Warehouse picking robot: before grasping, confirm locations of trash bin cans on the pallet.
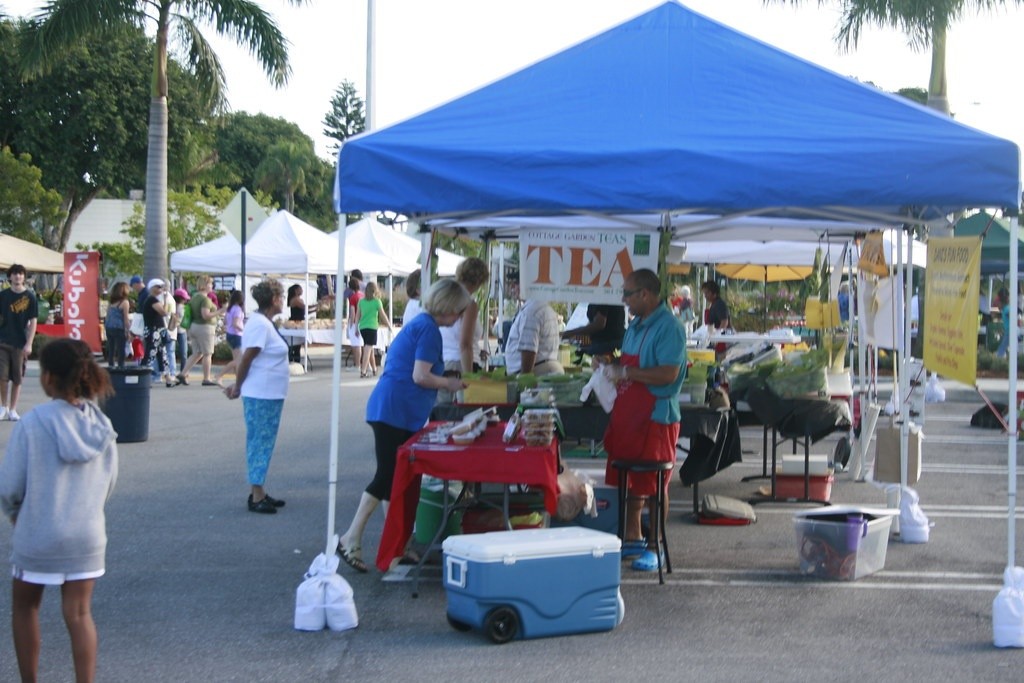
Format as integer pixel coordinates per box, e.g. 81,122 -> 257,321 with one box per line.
98,365 -> 155,443
415,472 -> 462,543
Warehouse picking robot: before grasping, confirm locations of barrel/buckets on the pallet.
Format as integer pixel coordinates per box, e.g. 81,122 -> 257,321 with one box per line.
415,479 -> 465,546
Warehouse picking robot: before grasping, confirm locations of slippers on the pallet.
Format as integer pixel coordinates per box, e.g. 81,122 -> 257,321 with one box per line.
337,540 -> 367,573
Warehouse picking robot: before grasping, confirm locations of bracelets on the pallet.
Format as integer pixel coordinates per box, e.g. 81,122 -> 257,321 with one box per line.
622,366 -> 627,379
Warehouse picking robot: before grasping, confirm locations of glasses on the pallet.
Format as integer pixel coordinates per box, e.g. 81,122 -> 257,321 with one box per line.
623,287 -> 643,297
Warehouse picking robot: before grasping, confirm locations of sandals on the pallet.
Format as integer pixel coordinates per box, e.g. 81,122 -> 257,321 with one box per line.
622,538 -> 647,559
632,548 -> 665,571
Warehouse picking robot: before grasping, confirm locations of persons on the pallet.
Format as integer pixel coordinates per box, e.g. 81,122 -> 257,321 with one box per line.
0,264 -> 39,421
0,338 -> 119,683
433,258 -> 488,407
504,272 -> 564,379
104,275 -> 306,387
223,279 -> 290,514
997,287 -> 1010,357
559,303 -> 626,364
702,281 -> 730,381
336,279 -> 471,572
403,269 -> 427,328
838,281 -> 849,322
591,269 -> 688,571
344,270 -> 366,298
355,282 -> 393,378
347,277 -> 365,371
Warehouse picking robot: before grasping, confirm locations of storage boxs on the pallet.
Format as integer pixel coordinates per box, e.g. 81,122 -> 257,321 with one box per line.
902,358 -> 925,413
873,424 -> 924,484
792,501 -> 902,581
442,525 -> 626,644
775,456 -> 835,503
553,458 -> 619,537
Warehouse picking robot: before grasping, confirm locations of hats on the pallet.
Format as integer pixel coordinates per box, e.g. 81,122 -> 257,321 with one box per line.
130,277 -> 141,286
148,279 -> 164,290
175,289 -> 191,300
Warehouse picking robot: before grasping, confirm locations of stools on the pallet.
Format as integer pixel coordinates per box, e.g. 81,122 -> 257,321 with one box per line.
610,457 -> 674,584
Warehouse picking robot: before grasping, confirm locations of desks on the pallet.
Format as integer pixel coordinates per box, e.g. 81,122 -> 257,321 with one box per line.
684,334 -> 801,343
379,423 -> 558,598
743,392 -> 851,506
279,326 -> 403,368
429,402 -> 742,514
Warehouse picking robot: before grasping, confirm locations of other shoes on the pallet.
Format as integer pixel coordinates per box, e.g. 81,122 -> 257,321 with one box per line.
0,407 -> 10,420
201,382 -> 215,385
248,495 -> 277,514
373,370 -> 378,375
176,374 -> 188,385
8,410 -> 20,420
166,380 -> 177,388
361,373 -> 369,378
261,494 -> 285,506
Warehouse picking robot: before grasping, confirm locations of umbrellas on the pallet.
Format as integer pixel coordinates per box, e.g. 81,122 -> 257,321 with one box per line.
713,263 -> 813,332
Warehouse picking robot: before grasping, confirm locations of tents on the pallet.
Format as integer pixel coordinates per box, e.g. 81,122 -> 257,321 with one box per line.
170,210 -> 468,373
915,212 -> 1024,349
326,0 -> 1020,567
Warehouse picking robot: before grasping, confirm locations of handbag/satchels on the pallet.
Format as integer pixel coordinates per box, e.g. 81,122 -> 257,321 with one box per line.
125,342 -> 134,358
131,336 -> 144,360
181,304 -> 191,330
